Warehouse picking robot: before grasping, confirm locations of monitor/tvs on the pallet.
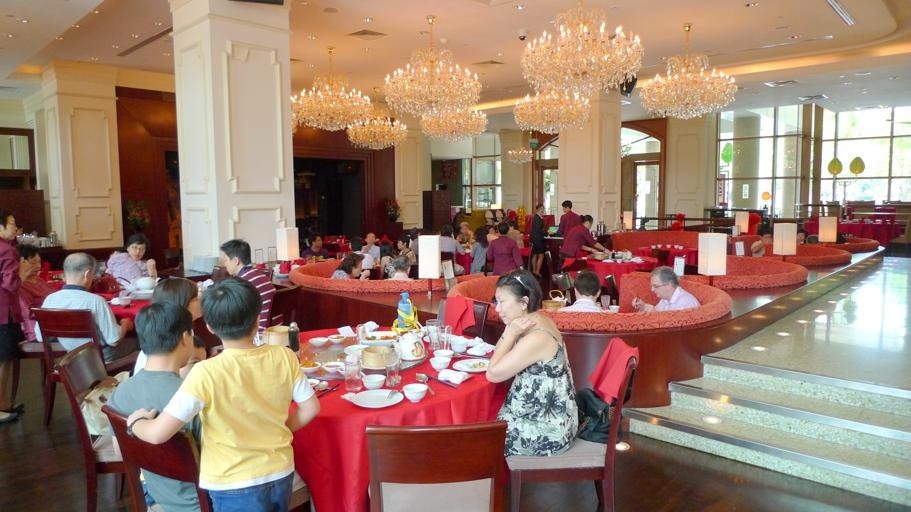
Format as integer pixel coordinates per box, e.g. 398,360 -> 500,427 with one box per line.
548,226 -> 559,235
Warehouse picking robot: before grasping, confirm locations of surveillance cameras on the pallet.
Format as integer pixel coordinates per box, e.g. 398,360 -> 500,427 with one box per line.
519,29 -> 528,40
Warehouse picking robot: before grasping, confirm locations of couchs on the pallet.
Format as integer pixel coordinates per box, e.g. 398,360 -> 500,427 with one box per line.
289,231 -> 880,334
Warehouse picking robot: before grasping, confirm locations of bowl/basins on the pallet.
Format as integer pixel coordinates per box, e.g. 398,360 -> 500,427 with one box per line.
329,334 -> 348,343
402,384 -> 429,403
309,338 -> 329,346
433,350 -> 456,361
430,357 -> 450,369
301,361 -> 345,376
362,374 -> 384,388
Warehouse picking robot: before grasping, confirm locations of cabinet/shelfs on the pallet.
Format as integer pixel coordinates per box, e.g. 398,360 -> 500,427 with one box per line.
423,191 -> 451,230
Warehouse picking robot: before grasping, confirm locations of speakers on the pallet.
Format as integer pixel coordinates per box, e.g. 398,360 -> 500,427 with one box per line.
620,76 -> 637,96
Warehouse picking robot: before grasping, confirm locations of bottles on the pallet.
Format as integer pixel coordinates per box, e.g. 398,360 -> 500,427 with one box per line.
18,231 -> 58,245
398,292 -> 416,328
288,322 -> 301,351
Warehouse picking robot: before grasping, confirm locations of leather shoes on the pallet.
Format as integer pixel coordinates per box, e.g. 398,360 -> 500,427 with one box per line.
0,401 -> 26,423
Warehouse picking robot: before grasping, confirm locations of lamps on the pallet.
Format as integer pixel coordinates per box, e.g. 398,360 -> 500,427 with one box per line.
828,157 -> 865,217
511,2 -> 645,135
638,23 -> 739,121
719,143 -> 733,169
382,15 -> 489,143
292,47 -> 406,150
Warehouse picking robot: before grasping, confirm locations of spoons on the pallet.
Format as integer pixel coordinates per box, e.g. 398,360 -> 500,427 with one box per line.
416,372 -> 437,396
314,381 -> 329,394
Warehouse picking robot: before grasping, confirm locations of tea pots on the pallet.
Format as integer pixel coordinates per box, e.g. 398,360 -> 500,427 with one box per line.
134,276 -> 161,289
550,289 -> 568,306
393,330 -> 427,360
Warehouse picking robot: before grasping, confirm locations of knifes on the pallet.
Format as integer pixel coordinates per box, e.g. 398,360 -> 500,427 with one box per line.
431,375 -> 458,388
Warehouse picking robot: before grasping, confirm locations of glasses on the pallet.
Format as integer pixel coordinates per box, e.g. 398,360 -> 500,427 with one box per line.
650,282 -> 669,289
498,271 -> 530,293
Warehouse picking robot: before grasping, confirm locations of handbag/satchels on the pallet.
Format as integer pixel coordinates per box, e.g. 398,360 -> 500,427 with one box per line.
576,387 -> 624,444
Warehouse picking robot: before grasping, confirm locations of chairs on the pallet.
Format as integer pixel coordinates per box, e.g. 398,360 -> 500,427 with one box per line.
504,337 -> 637,512
437,297 -> 490,339
365,420 -> 508,512
54,340 -> 126,512
27,306 -> 138,437
99,403 -> 312,512
259,267 -> 302,328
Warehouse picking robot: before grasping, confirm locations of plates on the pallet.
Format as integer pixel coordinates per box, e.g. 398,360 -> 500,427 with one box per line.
451,358 -> 491,374
308,378 -> 320,388
353,390 -> 403,409
345,331 -> 400,370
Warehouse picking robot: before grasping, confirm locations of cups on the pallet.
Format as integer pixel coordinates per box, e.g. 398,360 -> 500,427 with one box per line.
425,318 -> 494,355
255,332 -> 270,347
111,290 -> 154,306
596,249 -> 646,264
384,347 -> 403,387
597,295 -> 621,313
345,361 -> 363,390
